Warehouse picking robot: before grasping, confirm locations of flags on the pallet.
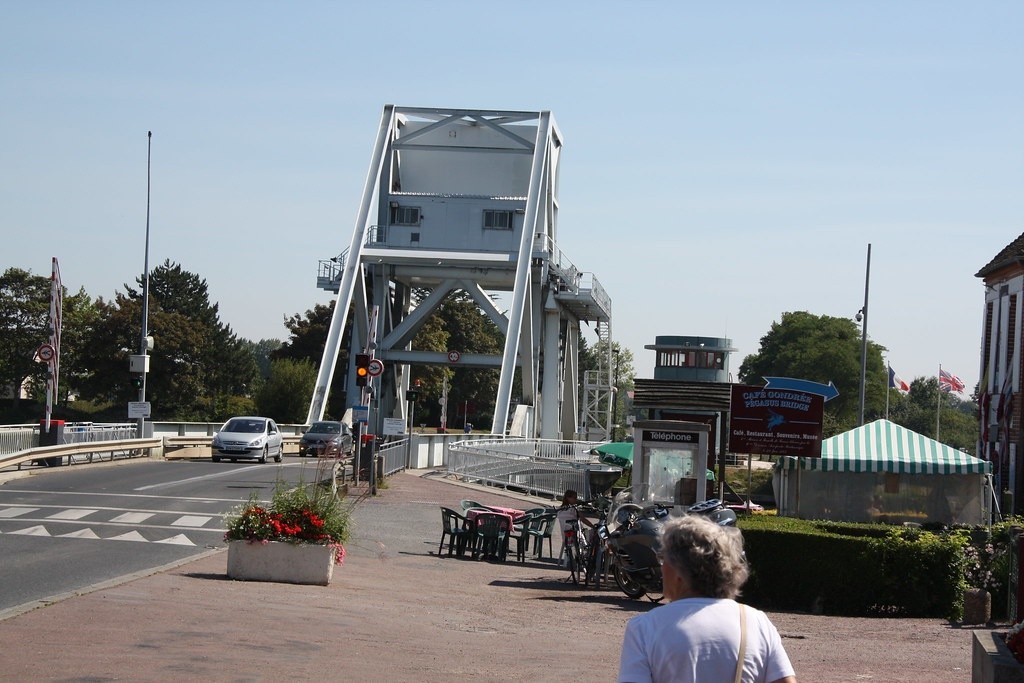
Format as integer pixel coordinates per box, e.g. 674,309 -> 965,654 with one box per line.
940,369 -> 966,394
888,365 -> 909,391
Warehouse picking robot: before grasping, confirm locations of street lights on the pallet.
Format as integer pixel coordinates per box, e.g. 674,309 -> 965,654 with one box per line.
855,244 -> 873,426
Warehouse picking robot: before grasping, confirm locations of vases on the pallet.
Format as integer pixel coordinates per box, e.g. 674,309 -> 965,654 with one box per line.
961,588 -> 991,623
228,539 -> 335,586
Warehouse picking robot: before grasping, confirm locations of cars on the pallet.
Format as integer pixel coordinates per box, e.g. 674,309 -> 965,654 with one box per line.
299,421 -> 353,457
211,416 -> 284,464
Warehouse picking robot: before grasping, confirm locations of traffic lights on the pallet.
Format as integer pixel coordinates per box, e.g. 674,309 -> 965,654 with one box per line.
355,354 -> 370,386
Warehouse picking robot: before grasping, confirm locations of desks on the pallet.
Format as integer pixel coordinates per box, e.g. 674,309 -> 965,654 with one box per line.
468,510 -> 514,557
485,505 -> 526,553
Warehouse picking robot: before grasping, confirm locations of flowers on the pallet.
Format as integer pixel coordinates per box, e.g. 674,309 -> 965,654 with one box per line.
959,543 -> 1009,593
218,447 -> 391,567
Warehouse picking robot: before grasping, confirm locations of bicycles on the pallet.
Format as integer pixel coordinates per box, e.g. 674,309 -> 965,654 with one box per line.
564,519 -> 589,586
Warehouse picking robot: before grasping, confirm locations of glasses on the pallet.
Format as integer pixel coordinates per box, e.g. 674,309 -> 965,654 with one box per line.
656,553 -> 665,565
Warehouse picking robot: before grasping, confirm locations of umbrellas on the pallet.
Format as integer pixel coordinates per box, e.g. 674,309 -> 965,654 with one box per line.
592,441 -> 716,482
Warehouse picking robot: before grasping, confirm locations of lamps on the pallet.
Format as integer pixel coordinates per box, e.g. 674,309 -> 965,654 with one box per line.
515,209 -> 524,214
390,201 -> 399,209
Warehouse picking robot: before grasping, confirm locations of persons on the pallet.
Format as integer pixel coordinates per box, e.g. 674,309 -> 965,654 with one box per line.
557,490 -> 597,585
855,493 -> 884,523
619,513 -> 798,683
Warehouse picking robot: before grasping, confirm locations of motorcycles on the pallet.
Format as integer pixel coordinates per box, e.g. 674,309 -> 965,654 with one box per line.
595,485 -> 738,599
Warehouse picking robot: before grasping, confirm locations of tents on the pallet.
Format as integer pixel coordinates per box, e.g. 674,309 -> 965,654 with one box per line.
771,418 -> 1005,539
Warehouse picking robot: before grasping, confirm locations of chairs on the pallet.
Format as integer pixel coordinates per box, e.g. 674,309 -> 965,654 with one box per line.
438,498 -> 561,564
